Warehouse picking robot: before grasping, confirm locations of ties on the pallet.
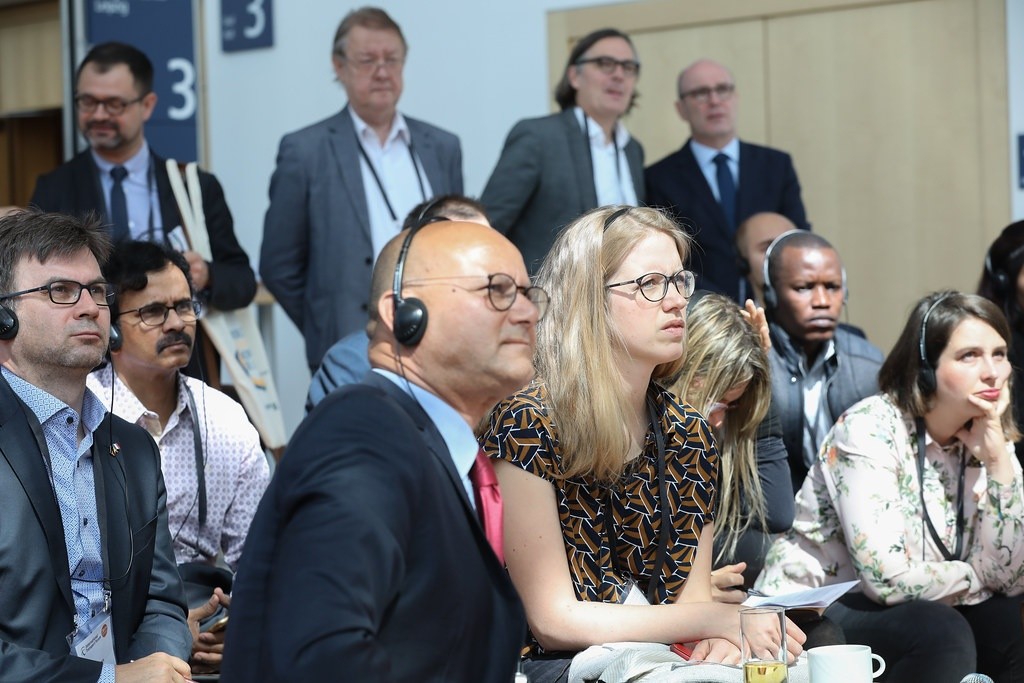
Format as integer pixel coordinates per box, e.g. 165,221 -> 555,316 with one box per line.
471,445 -> 505,570
110,167 -> 132,247
711,154 -> 736,243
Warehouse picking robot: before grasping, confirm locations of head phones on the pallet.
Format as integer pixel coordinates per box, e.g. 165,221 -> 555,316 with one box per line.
109,324 -> 123,351
763,229 -> 850,306
917,295 -> 954,395
392,215 -> 450,346
0,304 -> 19,340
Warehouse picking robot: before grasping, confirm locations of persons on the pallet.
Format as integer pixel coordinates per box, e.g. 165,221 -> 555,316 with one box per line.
86,240 -> 270,674
303,194 -> 489,420
643,60 -> 811,308
478,205 -> 807,683
259,7 -> 463,378
219,222 -> 551,683
478,29 -> 647,284
0,206 -> 203,683
650,210 -> 1024,682
32,41 -> 257,386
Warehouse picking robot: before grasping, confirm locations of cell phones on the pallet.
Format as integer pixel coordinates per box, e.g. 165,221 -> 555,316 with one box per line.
207,616 -> 229,633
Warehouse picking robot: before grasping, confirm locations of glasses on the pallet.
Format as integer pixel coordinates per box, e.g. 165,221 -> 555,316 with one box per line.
681,83 -> 735,101
605,270 -> 697,303
0,280 -> 117,306
405,273 -> 551,323
74,92 -> 144,116
343,55 -> 403,78
118,300 -> 205,326
577,56 -> 641,78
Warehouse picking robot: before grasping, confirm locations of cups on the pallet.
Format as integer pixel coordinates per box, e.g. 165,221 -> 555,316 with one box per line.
738,606 -> 789,682
807,644 -> 885,683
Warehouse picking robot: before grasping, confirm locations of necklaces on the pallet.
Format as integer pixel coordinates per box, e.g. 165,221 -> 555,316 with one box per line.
915,416 -> 973,562
